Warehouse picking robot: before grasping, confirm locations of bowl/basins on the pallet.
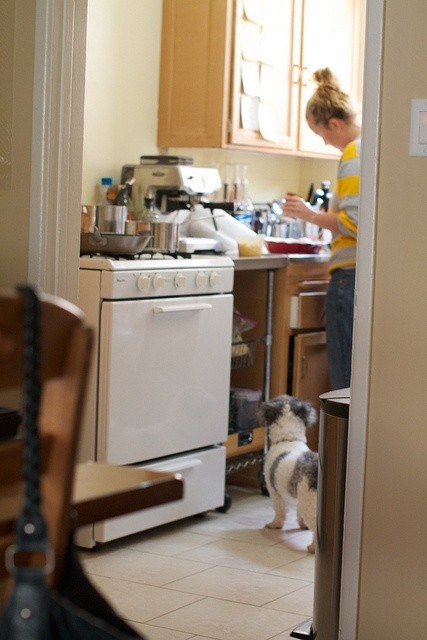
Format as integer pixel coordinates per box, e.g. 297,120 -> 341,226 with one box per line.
236,232 -> 264,257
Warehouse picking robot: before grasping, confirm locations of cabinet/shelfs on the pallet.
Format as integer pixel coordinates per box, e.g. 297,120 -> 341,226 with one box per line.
233,256 -> 335,487
157,1 -> 366,162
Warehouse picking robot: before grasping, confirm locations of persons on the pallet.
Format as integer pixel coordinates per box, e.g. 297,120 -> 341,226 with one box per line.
280,64 -> 362,390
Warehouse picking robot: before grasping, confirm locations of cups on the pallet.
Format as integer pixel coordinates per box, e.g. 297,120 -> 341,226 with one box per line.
98,205 -> 127,234
153,222 -> 179,252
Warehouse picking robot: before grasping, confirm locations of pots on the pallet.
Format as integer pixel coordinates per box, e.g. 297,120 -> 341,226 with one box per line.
81,227 -> 153,256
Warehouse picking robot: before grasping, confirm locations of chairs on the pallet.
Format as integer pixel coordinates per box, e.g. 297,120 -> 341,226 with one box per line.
2,285 -> 95,638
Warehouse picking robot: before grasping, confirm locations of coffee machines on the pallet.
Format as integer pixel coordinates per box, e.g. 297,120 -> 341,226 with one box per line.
121,155 -> 224,255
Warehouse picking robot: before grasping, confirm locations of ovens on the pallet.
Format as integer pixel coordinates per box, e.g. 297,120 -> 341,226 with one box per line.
77,292 -> 235,549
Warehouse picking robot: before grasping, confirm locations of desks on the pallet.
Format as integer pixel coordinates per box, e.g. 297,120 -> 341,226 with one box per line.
0,459 -> 182,640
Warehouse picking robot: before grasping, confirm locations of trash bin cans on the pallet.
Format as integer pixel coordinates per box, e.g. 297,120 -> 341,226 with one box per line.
307,388 -> 351,640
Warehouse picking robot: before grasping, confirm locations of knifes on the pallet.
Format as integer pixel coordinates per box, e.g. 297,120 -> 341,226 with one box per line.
305,178 -> 329,211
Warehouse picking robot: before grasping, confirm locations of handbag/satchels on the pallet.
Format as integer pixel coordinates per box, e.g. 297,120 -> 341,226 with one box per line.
0,540 -> 143,640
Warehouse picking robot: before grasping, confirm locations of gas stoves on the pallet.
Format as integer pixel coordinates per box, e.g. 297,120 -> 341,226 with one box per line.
78,250 -> 236,298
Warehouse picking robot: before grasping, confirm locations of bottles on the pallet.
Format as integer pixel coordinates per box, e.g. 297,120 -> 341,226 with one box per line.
232,164 -> 254,232
96,176 -> 117,205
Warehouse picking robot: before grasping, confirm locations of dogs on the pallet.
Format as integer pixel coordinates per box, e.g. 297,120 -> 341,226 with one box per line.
259,394 -> 321,555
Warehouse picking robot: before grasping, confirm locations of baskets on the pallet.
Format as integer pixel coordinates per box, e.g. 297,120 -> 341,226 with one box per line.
231,339 -> 255,369
